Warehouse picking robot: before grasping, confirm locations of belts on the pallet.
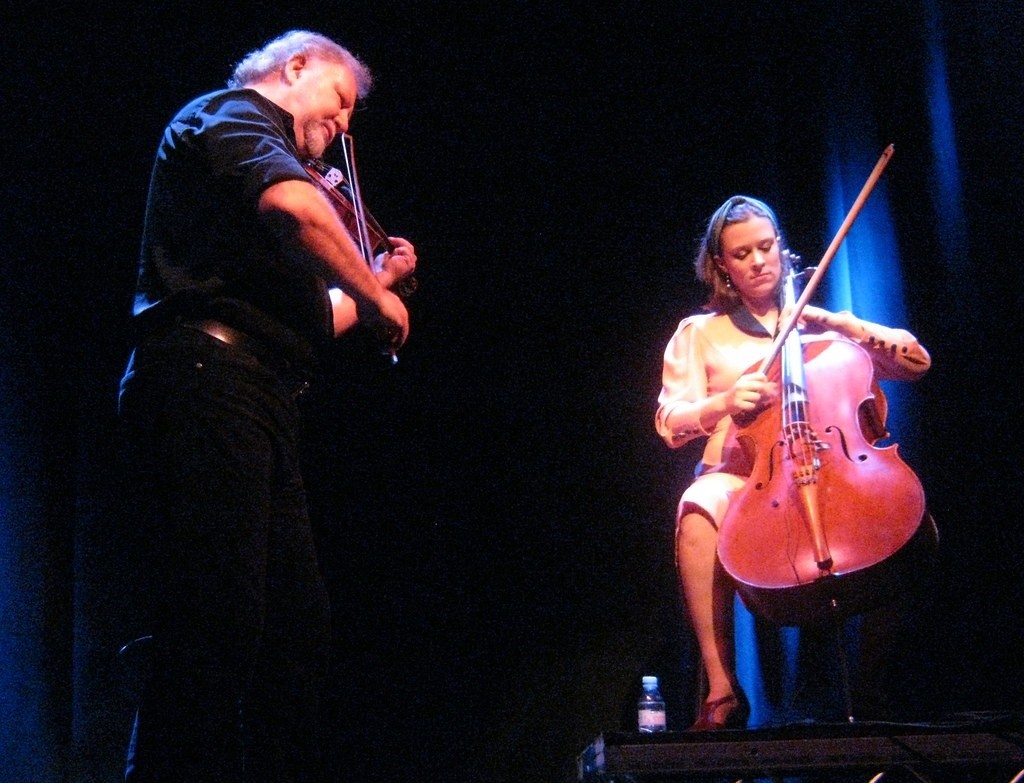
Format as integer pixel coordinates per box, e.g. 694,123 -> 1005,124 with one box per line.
186,319 -> 310,401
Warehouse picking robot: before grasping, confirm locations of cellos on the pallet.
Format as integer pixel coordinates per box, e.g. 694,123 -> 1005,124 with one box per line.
713,237 -> 939,721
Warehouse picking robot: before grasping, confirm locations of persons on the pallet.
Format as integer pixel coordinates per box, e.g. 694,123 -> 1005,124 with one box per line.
116,28 -> 417,783
655,195 -> 931,733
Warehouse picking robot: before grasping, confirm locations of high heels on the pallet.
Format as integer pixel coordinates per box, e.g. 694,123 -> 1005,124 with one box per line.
683,688 -> 751,732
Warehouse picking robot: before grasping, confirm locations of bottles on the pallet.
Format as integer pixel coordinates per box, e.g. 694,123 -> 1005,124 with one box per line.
637,676 -> 666,733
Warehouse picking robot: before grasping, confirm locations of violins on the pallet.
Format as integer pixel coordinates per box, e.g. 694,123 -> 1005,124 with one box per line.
306,162 -> 419,296
